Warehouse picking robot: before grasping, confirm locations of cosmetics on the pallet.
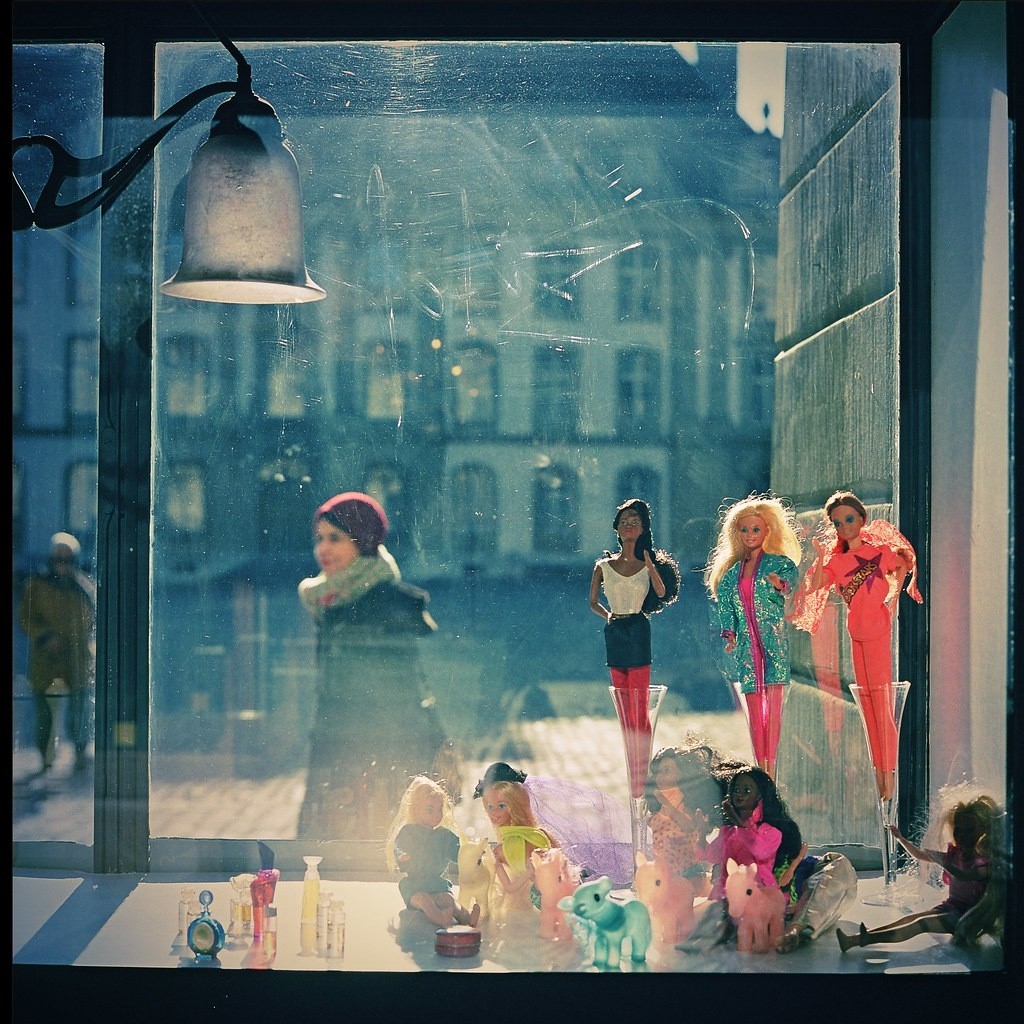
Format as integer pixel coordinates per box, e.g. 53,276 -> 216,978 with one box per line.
178,855 -> 346,959
435,925 -> 481,958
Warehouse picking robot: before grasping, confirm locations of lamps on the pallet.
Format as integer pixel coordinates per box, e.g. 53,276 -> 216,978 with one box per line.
1,1 -> 326,305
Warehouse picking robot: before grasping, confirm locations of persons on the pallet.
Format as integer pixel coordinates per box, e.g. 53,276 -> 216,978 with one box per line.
834,795 -> 1005,953
703,494 -> 802,782
786,492 -> 924,802
473,763 -> 561,910
297,492 -> 438,839
386,776 -> 480,928
587,498 -> 681,797
645,746 -> 857,954
21,532 -> 96,771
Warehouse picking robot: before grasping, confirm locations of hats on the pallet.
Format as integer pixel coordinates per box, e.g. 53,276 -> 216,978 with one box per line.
315,493 -> 388,556
